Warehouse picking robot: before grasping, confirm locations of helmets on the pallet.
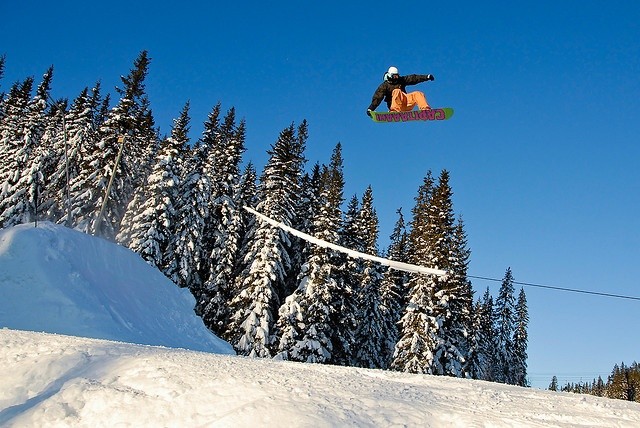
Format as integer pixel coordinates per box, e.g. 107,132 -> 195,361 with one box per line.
388,66 -> 398,75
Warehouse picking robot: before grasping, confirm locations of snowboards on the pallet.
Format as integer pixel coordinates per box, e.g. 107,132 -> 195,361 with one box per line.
366,108 -> 454,122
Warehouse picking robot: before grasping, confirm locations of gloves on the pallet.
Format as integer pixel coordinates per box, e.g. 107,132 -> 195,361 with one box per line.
428,74 -> 434,80
367,109 -> 372,116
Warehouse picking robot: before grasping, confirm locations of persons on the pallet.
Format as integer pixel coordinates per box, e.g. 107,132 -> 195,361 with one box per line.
366,65 -> 434,116
